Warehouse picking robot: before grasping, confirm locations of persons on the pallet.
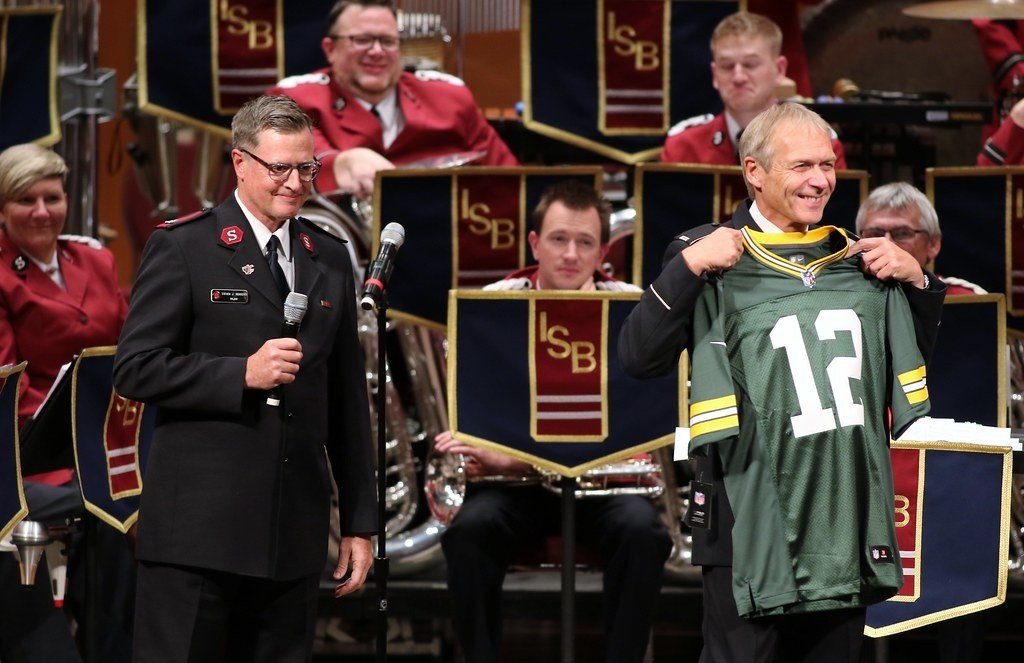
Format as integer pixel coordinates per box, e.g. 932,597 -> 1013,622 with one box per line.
971,18 -> 1024,569
434,187 -> 675,663
857,183 -> 987,295
0,143 -> 136,663
264,0 -> 520,526
620,102 -> 946,663
661,12 -> 848,170
112,95 -> 377,663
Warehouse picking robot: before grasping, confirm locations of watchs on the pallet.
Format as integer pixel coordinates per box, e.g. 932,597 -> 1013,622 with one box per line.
922,275 -> 930,290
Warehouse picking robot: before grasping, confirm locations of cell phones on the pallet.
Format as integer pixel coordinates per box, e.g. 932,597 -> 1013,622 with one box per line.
360,221 -> 405,310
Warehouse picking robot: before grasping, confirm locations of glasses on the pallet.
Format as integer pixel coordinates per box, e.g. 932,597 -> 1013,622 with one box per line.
859,226 -> 930,244
241,148 -> 322,182
329,34 -> 403,53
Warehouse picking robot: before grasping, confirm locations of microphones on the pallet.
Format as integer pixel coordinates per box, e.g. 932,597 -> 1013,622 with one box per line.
265,292 -> 308,406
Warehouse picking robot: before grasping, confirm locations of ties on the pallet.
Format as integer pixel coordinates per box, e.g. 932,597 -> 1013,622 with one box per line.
737,129 -> 744,142
266,235 -> 290,303
370,108 -> 379,120
